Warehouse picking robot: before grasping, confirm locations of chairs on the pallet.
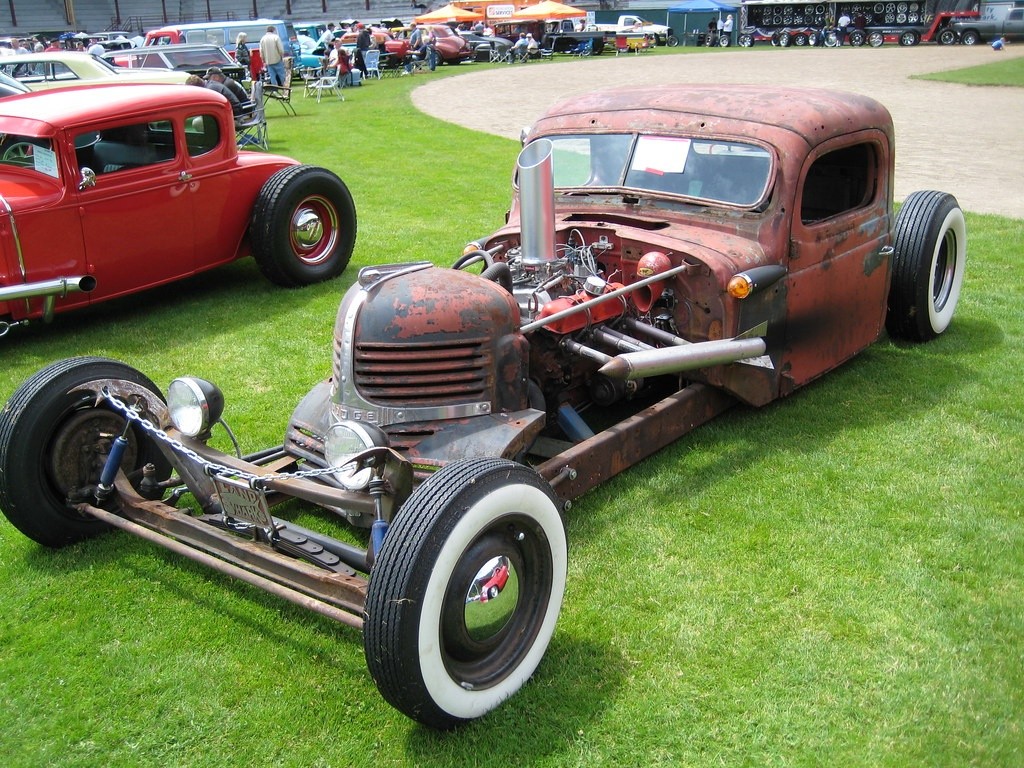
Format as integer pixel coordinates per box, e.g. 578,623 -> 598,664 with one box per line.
615,35 -> 632,54
300,43 -> 433,104
488,36 -> 556,64
263,57 -> 297,116
91,141 -> 156,173
570,39 -> 585,58
234,80 -> 269,152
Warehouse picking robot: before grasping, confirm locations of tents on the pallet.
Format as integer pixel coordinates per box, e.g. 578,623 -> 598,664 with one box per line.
60,31 -> 107,46
414,5 -> 483,27
512,1 -> 587,30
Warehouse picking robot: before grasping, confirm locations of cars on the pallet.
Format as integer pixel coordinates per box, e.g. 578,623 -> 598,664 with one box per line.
0,79 -> 967,727
0,82 -> 357,329
0,16 -> 514,98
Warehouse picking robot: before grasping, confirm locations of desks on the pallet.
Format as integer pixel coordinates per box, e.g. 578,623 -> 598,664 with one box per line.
406,50 -> 422,71
379,51 -> 397,77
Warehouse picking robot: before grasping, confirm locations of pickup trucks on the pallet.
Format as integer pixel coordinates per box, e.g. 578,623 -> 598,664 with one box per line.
953,7 -> 1024,45
545,19 -> 617,55
587,15 -> 671,44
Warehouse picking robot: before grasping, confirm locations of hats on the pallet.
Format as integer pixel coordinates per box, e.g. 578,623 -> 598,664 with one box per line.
50,38 -> 58,43
202,66 -> 222,78
328,23 -> 336,27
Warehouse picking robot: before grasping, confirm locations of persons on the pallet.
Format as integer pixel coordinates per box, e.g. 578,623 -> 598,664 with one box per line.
0,38 -> 104,76
401,22 -> 427,75
642,33 -> 660,46
992,37 -> 1005,50
185,67 -> 252,120
508,33 -> 538,64
575,20 -> 585,32
235,26 -> 286,94
458,20 -> 493,38
819,12 -> 866,46
708,15 -> 734,47
514,22 -> 540,36
427,27 -> 436,71
312,23 -> 377,88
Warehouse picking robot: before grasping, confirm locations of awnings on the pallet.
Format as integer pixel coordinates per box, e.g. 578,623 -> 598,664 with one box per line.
666,0 -> 738,48
